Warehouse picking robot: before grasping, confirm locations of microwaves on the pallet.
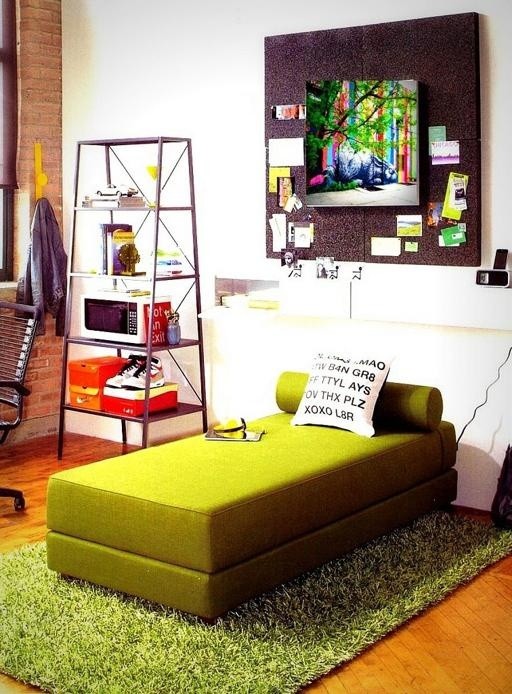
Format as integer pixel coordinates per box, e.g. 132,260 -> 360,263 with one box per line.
78,291 -> 170,345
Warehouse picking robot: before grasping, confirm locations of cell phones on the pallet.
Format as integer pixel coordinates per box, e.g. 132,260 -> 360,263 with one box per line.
493,249 -> 508,269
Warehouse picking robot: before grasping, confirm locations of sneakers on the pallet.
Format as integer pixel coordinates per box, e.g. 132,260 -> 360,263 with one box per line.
122,356 -> 167,390
105,355 -> 148,388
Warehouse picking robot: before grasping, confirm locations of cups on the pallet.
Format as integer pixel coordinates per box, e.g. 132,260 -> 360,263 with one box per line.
167,324 -> 180,344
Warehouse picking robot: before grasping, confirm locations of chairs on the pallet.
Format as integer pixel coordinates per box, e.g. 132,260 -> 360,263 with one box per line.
0,300 -> 40,516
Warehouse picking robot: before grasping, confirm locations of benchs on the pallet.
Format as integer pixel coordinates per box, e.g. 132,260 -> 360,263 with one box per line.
45,370 -> 460,622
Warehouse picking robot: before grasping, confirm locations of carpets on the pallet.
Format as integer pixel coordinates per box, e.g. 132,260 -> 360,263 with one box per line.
0,505 -> 512,694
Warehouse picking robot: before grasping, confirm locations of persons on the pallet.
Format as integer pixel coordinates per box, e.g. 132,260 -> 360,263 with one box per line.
316,263 -> 328,278
455,181 -> 466,199
282,251 -> 296,277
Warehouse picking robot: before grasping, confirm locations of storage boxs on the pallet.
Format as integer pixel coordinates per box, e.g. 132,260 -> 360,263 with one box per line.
68,353 -> 183,419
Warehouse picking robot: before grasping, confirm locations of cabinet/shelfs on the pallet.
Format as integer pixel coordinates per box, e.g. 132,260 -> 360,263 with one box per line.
55,135 -> 209,462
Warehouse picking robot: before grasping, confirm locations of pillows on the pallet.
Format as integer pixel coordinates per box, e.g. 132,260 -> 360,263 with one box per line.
292,347 -> 393,439
